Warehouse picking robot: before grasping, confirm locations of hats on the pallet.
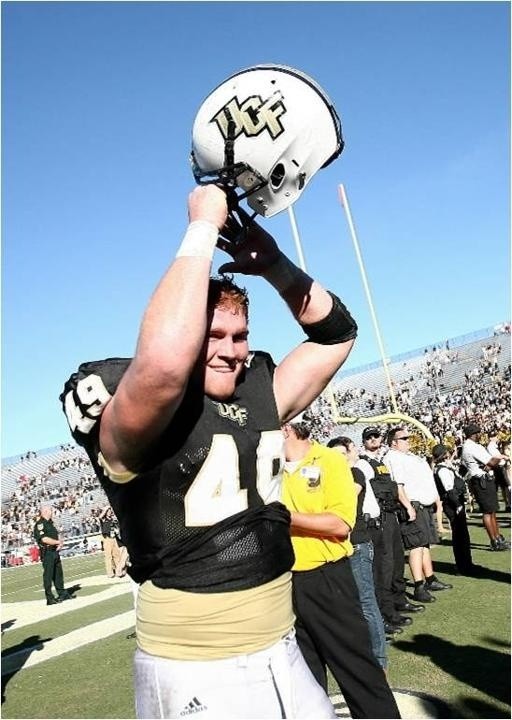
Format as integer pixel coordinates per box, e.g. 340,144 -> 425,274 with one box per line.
431,444 -> 449,461
362,426 -> 379,437
488,431 -> 498,437
463,424 -> 481,440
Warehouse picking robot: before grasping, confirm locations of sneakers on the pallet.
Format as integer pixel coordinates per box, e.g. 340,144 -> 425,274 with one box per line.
425,579 -> 453,592
382,614 -> 404,634
108,571 -> 127,578
47,598 -> 62,605
396,616 -> 413,627
490,534 -> 511,551
59,594 -> 77,601
395,602 -> 426,613
414,589 -> 437,603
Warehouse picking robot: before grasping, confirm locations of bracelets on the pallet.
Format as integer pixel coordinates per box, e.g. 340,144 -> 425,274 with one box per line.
175,221 -> 219,262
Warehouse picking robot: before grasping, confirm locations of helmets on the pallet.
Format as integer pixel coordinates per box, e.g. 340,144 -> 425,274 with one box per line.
188,62 -> 346,222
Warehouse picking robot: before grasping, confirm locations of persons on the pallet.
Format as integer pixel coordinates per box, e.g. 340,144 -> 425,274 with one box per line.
279,414 -> 401,719
279,322 -> 511,646
2,440 -> 133,584
34,506 -> 75,606
324,438 -> 388,684
62,181 -> 358,720
